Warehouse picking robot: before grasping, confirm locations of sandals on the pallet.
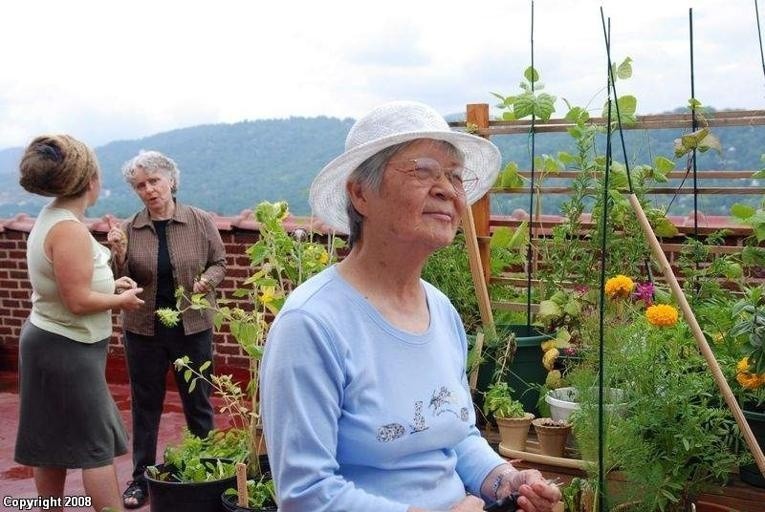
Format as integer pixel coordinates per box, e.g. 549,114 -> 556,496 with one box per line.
122,481 -> 148,509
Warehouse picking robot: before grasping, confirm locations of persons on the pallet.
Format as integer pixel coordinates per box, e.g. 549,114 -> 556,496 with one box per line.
106,151 -> 229,509
260,96 -> 562,511
13,133 -> 145,512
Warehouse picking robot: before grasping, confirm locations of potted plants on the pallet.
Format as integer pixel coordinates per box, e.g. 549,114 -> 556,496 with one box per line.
143,55 -> 765,512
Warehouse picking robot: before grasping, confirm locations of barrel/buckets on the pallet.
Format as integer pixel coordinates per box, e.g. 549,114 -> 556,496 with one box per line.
545,387 -> 630,448
143,458 -> 238,512
466,323 -> 557,426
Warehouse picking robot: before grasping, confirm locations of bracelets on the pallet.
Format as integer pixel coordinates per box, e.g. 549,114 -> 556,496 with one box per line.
488,465 -> 510,498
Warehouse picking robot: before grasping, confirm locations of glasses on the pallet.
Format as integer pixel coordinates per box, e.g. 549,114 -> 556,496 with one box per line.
386,157 -> 479,195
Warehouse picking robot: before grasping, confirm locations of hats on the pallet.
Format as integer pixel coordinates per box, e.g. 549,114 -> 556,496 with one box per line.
309,102 -> 501,235
